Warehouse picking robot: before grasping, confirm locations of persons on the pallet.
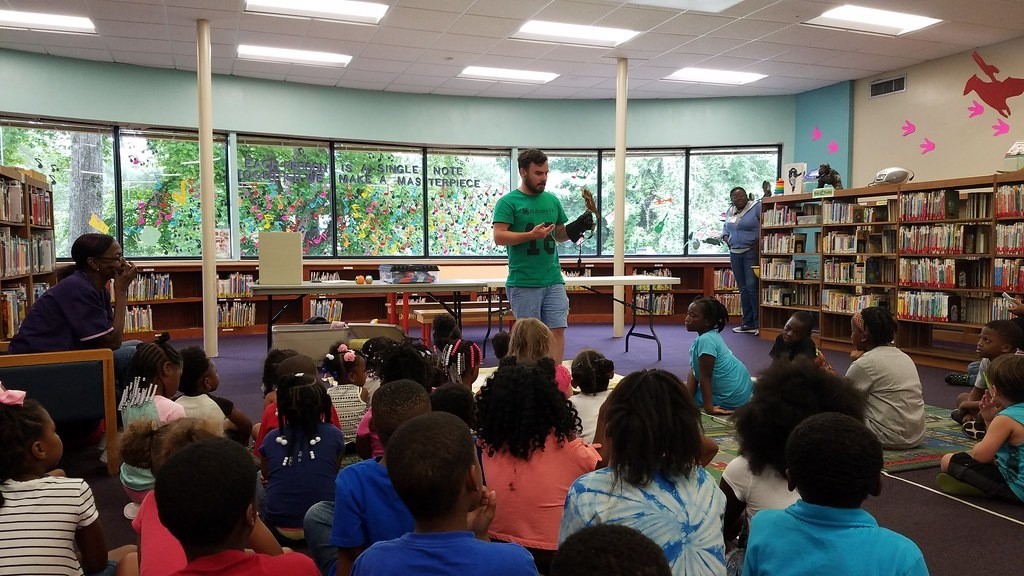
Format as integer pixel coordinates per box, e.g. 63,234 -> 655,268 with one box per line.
8,232 -> 142,432
493,150 -> 593,365
717,355 -> 866,546
721,180 -> 772,335
722,412 -> 929,576
0,296 -> 753,576
936,301 -> 1024,503
844,306 -> 927,451
768,311 -> 841,379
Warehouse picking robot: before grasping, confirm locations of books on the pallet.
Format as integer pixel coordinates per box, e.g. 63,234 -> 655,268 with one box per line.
632,268 -> 675,315
378,264 -> 440,273
762,183 -> 1024,322
110,273 -> 174,333
715,270 -> 744,316
309,271 -> 343,323
0,177 -> 57,339
215,271 -> 256,327
398,296 -> 427,320
561,268 -> 592,291
477,295 -> 500,300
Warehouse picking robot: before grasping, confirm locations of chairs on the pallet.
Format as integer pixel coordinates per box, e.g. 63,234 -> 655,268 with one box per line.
0,342 -> 119,476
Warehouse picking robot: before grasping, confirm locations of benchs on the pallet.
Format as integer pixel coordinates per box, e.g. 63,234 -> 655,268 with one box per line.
385,301 -> 518,349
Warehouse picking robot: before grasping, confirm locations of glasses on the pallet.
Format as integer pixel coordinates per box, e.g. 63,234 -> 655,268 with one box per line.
94,252 -> 123,262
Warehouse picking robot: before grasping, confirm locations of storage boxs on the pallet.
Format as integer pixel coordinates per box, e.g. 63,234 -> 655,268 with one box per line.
259,232 -> 303,285
271,325 -> 353,367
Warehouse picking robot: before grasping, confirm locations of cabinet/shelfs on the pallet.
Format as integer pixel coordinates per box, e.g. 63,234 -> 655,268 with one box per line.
757,170 -> 1024,371
106,264 -> 517,343
561,262 -> 744,325
0,164 -> 58,353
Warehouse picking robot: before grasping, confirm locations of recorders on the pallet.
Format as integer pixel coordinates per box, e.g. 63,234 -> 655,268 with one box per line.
874,166 -> 909,185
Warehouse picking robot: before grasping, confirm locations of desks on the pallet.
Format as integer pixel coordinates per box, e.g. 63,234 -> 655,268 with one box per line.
246,275 -> 681,361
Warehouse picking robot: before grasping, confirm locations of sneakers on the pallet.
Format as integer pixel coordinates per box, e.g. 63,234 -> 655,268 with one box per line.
961,420 -> 987,440
945,372 -> 969,386
732,323 -> 759,336
950,408 -> 964,423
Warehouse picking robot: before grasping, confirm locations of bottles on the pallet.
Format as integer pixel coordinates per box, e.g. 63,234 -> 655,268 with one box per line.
355,275 -> 372,284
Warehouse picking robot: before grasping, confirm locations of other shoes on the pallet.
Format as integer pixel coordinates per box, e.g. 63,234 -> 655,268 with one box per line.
935,472 -> 992,498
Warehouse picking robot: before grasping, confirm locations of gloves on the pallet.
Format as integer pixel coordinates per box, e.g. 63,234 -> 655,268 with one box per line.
566,210 -> 595,243
